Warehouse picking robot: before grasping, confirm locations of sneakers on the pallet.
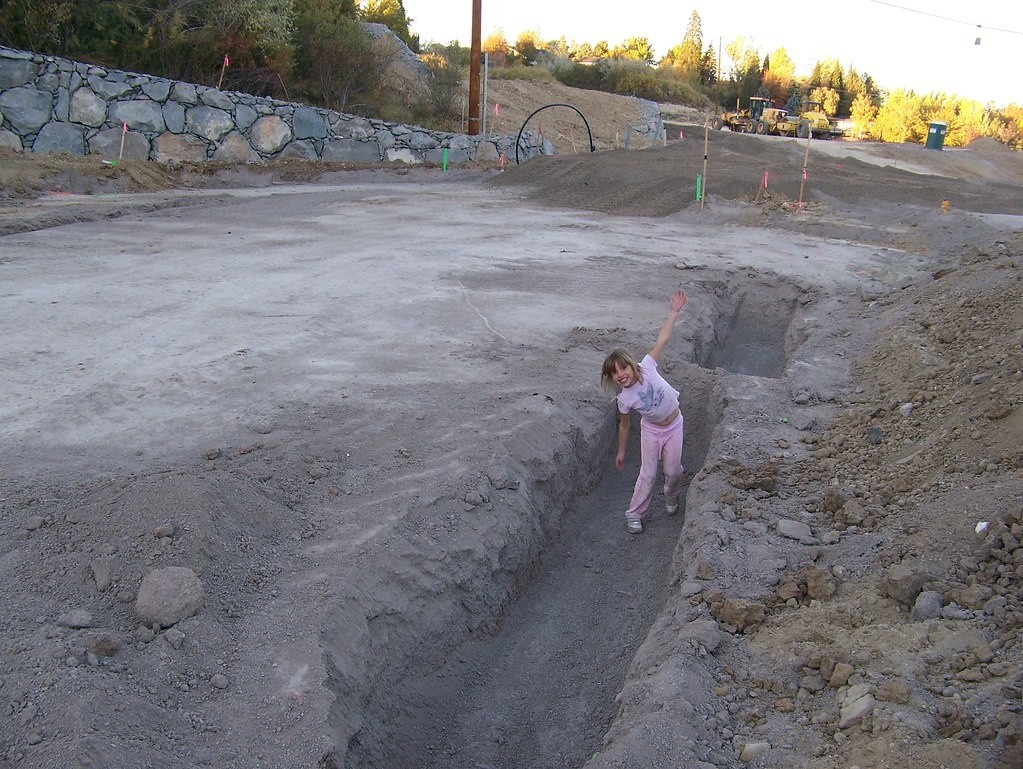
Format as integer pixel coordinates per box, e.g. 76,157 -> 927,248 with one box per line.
626,517 -> 643,534
665,494 -> 679,516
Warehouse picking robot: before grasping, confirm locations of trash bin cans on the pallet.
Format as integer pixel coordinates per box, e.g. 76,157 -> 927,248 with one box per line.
926,122 -> 947,151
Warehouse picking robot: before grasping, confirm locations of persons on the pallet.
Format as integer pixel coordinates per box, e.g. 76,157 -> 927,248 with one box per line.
600,289 -> 687,534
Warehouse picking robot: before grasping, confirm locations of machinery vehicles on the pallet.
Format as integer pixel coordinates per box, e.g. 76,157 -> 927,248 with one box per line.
712,96 -> 843,141
